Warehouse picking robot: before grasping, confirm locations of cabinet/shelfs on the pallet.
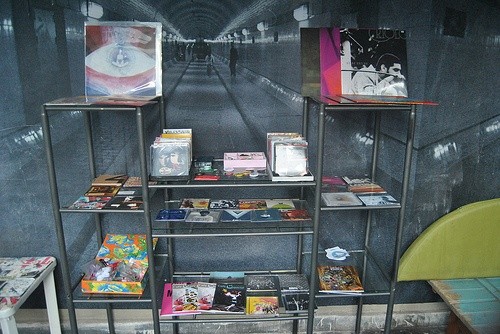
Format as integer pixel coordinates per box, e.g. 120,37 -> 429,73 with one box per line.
41,97 -> 417,334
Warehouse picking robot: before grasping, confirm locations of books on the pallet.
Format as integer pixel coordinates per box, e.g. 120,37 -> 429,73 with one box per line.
151,129 -> 192,177
70,174 -> 157,210
155,197 -> 313,223
269,132 -> 314,182
321,173 -> 400,208
160,273 -> 319,316
316,264 -> 365,293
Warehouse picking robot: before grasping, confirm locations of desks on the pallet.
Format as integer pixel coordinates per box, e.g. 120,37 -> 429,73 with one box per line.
428,276 -> 500,334
0,256 -> 62,334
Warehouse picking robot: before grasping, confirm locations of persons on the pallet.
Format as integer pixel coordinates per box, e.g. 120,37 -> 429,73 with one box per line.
193,211 -> 213,223
352,54 -> 408,97
229,41 -> 239,78
181,42 -> 192,57
